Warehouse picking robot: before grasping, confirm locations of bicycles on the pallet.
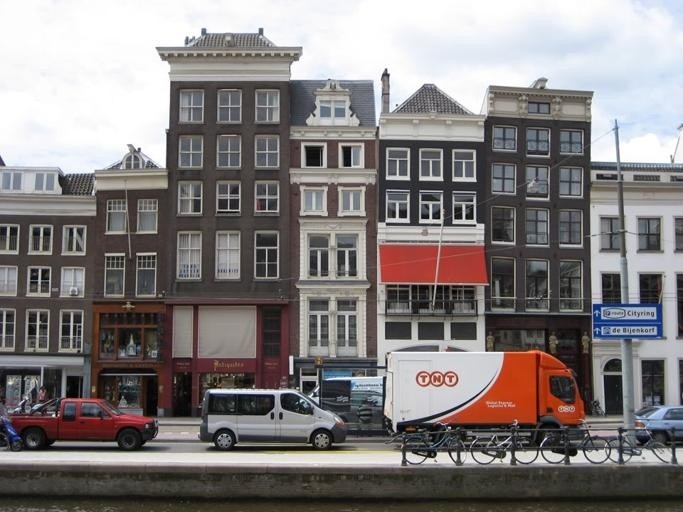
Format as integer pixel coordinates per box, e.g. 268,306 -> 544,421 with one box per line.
591,397 -> 606,416
397,420 -> 468,465
468,417 -> 540,465
603,421 -> 672,463
538,418 -> 611,464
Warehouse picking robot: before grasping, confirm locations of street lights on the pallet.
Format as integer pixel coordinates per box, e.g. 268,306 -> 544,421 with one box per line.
313,354 -> 324,404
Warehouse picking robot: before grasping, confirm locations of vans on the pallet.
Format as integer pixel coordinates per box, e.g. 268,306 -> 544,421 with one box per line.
293,375 -> 385,434
195,386 -> 347,451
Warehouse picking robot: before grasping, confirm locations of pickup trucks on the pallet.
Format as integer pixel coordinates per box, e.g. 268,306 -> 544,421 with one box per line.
7,396 -> 160,450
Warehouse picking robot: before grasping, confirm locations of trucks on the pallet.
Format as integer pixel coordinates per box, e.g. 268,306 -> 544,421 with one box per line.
381,350 -> 587,449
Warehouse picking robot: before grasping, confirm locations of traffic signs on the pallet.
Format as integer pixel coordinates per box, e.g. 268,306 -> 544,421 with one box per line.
592,324 -> 662,340
592,303 -> 662,325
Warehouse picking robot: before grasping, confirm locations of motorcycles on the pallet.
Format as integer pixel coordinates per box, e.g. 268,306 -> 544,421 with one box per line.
13,396 -> 50,415
0,415 -> 23,452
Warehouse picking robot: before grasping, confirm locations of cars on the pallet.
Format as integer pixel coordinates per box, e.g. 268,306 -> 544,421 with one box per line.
633,403 -> 683,446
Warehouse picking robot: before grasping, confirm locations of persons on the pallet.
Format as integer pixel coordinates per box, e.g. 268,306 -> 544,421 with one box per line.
548,330 -> 558,354
39,385 -> 47,404
213,397 -> 307,414
485,331 -> 496,352
582,331 -> 591,353
